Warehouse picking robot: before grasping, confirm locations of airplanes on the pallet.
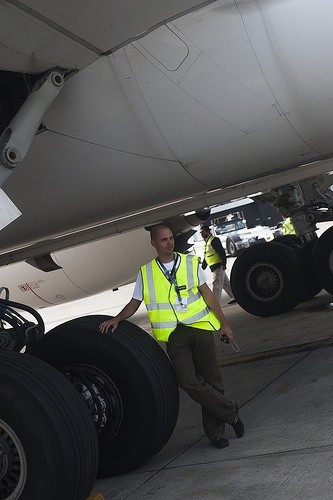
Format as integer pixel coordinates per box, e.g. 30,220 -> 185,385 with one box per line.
1,1 -> 332,499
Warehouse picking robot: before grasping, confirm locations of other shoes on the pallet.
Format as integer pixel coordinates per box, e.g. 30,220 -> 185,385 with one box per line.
209,436 -> 229,449
228,300 -> 236,304
231,402 -> 246,438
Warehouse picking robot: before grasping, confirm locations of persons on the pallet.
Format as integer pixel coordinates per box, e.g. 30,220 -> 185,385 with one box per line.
282,214 -> 297,235
201,226 -> 237,305
99,224 -> 244,448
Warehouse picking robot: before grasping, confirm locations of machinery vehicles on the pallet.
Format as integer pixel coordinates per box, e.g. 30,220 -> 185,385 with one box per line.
192,210 -> 272,262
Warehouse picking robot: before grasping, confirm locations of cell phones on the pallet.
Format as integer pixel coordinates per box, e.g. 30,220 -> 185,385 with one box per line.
221,335 -> 229,344
